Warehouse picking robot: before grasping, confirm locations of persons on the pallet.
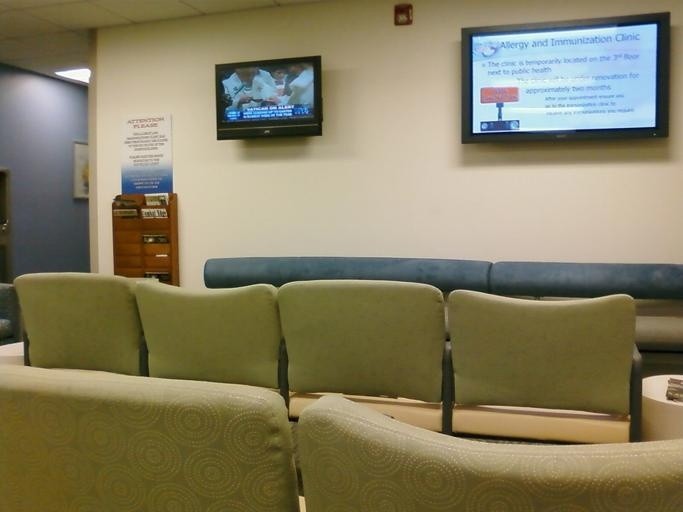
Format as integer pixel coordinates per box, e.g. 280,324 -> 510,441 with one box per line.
218,62 -> 314,121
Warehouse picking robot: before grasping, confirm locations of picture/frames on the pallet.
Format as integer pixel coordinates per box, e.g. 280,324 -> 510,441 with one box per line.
73,140 -> 88,200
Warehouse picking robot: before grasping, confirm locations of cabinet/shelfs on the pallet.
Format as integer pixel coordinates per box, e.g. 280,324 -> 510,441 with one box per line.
113,192 -> 179,286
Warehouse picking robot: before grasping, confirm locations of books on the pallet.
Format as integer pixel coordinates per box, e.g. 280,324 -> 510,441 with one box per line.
114,192 -> 171,281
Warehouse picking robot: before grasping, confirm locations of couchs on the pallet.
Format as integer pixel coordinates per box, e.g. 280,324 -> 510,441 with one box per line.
201,256 -> 489,373
490,259 -> 683,374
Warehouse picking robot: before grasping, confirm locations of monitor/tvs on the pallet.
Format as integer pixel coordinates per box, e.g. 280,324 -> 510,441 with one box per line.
460,11 -> 672,146
215,55 -> 323,140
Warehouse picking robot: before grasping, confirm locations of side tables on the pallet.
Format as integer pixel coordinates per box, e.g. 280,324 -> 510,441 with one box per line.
638,373 -> 683,445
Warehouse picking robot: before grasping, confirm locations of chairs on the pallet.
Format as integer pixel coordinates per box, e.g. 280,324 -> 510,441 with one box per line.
0,283 -> 21,345
1,363 -> 299,511
14,271 -> 147,379
277,277 -> 447,433
446,287 -> 642,445
294,392 -> 682,511
133,278 -> 284,390
